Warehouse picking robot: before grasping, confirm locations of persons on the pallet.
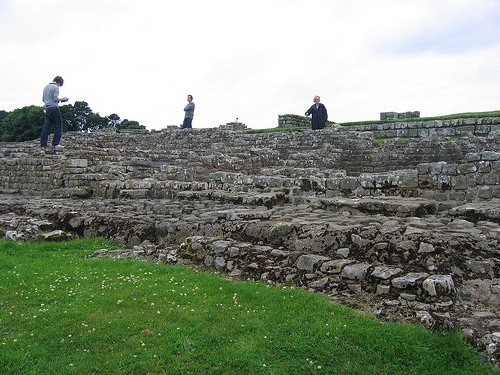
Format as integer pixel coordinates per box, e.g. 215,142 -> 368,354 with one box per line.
40,76 -> 69,154
305,96 -> 328,129
183,95 -> 195,128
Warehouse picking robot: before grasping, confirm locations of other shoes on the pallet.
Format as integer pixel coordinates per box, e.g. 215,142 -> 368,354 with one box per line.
52,145 -> 64,151
42,147 -> 50,152
